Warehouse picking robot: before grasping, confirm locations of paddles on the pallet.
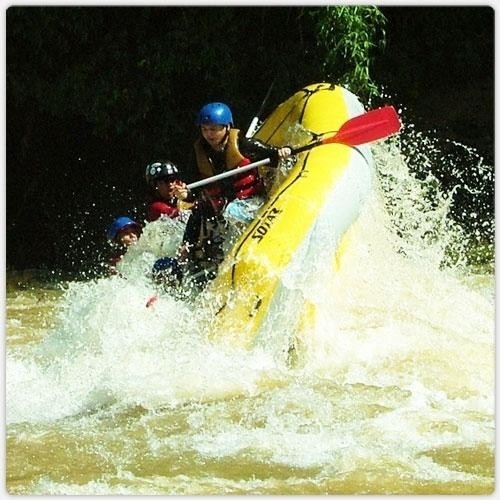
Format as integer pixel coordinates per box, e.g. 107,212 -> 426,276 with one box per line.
185,106 -> 401,190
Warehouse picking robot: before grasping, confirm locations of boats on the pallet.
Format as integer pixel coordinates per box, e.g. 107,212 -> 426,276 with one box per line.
107,77 -> 383,378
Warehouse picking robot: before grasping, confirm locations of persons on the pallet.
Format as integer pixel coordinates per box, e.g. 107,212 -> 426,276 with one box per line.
152,244 -> 203,303
107,217 -> 142,280
146,160 -> 180,222
169,103 -> 297,271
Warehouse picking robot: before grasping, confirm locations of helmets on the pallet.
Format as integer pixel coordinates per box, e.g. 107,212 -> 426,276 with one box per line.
195,101 -> 234,132
146,159 -> 179,191
107,216 -> 141,250
